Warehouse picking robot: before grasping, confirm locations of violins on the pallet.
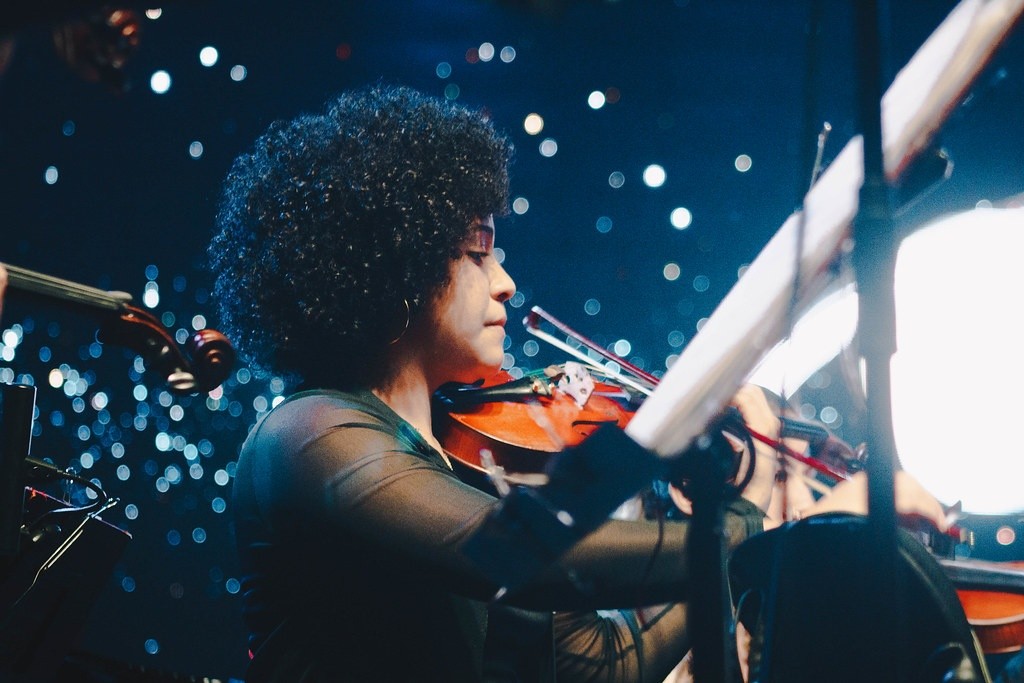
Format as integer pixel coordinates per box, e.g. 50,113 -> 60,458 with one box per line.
0,262 -> 238,393
425,362 -> 870,480
933,560 -> 1024,657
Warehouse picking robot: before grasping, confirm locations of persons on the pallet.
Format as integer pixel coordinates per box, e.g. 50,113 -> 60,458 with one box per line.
207,86 -> 947,683
